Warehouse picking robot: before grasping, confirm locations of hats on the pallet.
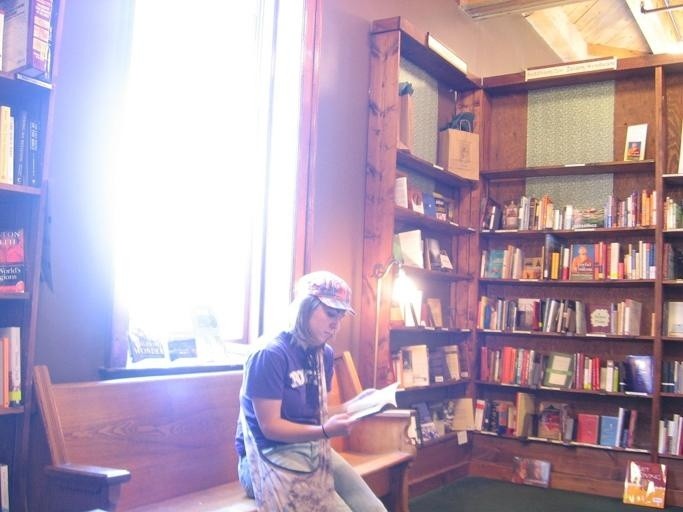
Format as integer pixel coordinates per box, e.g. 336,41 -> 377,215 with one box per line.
300,272 -> 357,316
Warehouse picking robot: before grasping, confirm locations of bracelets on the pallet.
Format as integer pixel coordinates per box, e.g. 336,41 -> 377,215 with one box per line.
321,424 -> 330,439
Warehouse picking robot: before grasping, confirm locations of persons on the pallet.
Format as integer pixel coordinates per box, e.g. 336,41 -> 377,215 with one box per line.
627,143 -> 640,158
238,269 -> 388,512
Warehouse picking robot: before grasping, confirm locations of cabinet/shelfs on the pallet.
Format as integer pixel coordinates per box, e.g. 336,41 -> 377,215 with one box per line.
0,0 -> 66,512
346,16 -> 471,499
472,51 -> 683,508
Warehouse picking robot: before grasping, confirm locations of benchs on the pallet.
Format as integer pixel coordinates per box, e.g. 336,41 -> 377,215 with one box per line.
32,351 -> 417,512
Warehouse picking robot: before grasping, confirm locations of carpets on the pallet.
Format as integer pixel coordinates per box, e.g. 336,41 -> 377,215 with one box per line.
408,474 -> 683,511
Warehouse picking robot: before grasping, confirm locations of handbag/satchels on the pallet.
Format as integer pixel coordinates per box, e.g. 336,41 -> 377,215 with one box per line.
244,426 -> 334,512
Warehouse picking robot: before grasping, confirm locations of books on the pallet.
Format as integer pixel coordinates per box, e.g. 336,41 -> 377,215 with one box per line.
0,1 -> 53,512
395,230 -> 454,274
621,459 -> 667,510
476,295 -> 643,336
394,177 -> 456,225
479,345 -> 652,396
394,343 -> 459,388
480,189 -> 655,230
479,234 -> 657,279
510,457 -> 549,489
392,289 -> 441,328
475,393 -> 638,448
345,381 -> 400,423
658,413 -> 682,457
662,299 -> 682,337
663,243 -> 677,279
661,196 -> 681,229
661,359 -> 682,394
409,398 -> 474,440
623,123 -> 648,163
